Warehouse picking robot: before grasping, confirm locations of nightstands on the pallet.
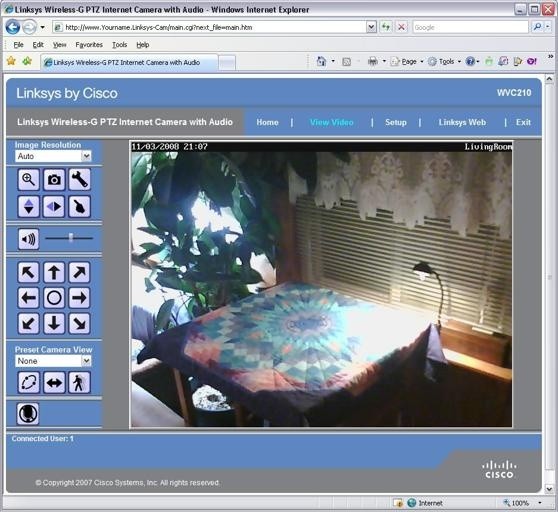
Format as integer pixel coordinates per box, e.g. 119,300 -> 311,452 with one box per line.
440,318 -> 512,387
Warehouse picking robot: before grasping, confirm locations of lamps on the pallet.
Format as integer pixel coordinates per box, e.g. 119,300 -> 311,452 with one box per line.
412,260 -> 443,335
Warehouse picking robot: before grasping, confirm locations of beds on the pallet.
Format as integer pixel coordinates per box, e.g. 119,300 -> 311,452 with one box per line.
136,281 -> 448,427
